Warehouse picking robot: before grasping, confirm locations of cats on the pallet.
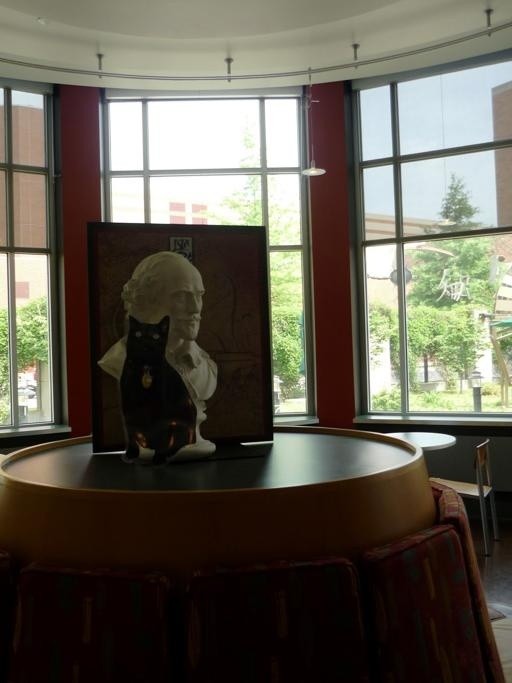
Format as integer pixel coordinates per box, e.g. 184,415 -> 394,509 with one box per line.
117,312 -> 198,467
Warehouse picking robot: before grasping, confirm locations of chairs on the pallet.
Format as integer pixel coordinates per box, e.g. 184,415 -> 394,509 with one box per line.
428,438 -> 499,557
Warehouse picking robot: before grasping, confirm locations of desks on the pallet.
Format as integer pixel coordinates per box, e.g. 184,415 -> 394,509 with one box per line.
383,432 -> 457,477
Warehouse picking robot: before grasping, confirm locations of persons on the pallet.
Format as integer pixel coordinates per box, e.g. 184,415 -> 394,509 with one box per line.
96,247 -> 218,459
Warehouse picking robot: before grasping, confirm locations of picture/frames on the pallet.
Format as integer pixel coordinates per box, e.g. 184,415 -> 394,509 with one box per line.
86,221 -> 273,453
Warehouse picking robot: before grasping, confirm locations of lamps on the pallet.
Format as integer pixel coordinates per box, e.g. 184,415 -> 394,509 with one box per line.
301,67 -> 327,176
